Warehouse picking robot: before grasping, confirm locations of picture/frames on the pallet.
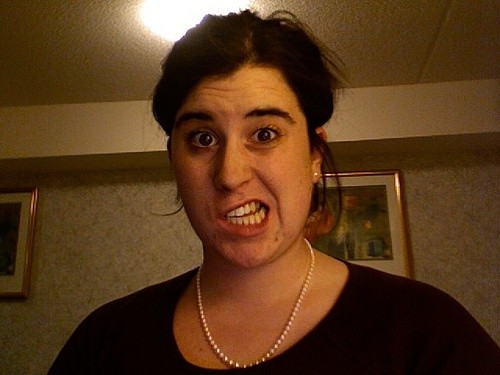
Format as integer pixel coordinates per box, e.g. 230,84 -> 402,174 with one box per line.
304,168 -> 414,280
0,186 -> 39,300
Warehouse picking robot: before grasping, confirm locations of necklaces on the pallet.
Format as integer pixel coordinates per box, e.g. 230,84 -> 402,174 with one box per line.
196,238 -> 315,369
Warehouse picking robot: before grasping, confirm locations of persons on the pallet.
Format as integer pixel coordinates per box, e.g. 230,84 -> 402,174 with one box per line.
316,191 -> 392,260
47,10 -> 500,375
